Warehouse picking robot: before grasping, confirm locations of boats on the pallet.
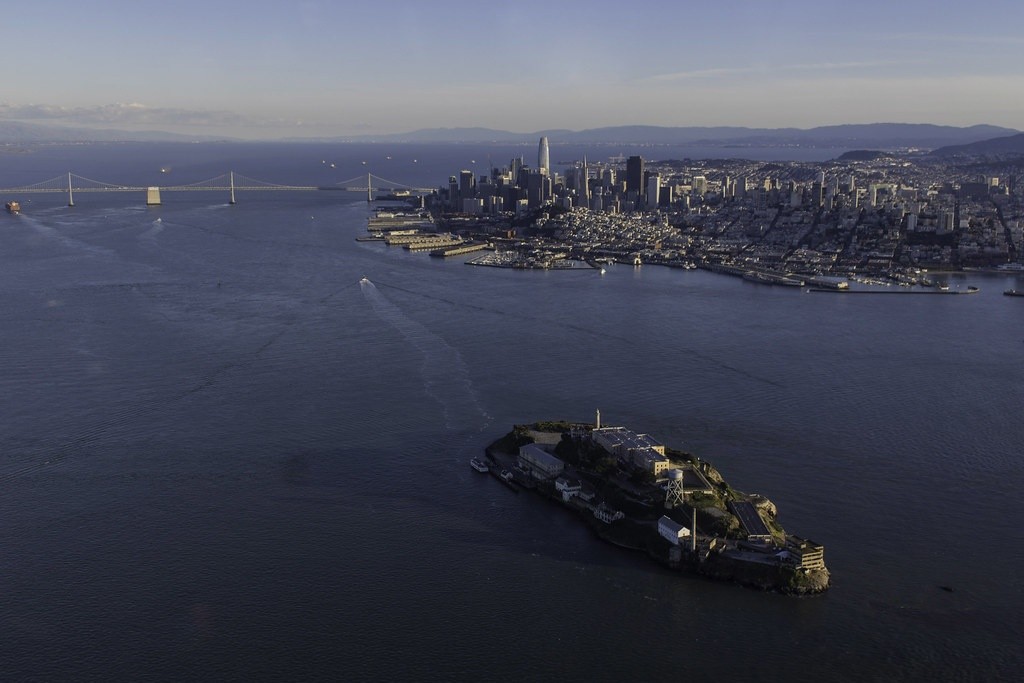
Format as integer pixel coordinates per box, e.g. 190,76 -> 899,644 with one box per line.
471,406 -> 829,594
1004,288 -> 1023,296
